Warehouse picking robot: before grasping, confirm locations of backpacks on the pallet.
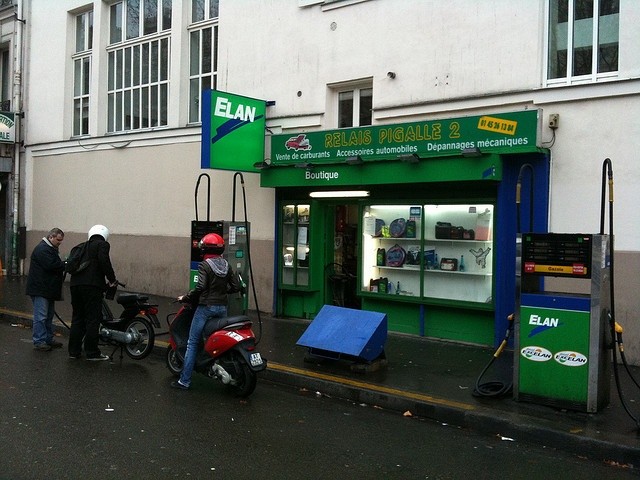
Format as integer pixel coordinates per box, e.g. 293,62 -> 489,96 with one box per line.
64,241 -> 95,275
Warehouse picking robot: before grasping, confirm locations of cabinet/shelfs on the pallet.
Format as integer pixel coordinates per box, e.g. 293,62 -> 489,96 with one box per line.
359,202 -> 493,308
278,203 -> 310,290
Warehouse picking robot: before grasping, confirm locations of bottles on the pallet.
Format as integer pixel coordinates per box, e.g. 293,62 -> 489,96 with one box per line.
459,255 -> 465,272
396,281 -> 401,294
387,281 -> 391,294
433,253 -> 439,269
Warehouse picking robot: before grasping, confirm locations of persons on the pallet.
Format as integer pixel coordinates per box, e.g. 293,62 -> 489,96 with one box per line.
170,233 -> 233,391
23,226 -> 66,351
66,224 -> 118,362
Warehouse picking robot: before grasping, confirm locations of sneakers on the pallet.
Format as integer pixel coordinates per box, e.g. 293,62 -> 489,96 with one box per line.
33,341 -> 52,351
85,350 -> 110,361
46,339 -> 64,349
170,378 -> 190,391
69,351 -> 84,360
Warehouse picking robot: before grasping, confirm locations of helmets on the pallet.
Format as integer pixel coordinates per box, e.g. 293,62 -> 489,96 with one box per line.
198,234 -> 226,256
88,224 -> 110,241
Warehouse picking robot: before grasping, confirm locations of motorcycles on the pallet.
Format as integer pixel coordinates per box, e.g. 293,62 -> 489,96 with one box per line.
97,281 -> 162,361
165,297 -> 267,397
